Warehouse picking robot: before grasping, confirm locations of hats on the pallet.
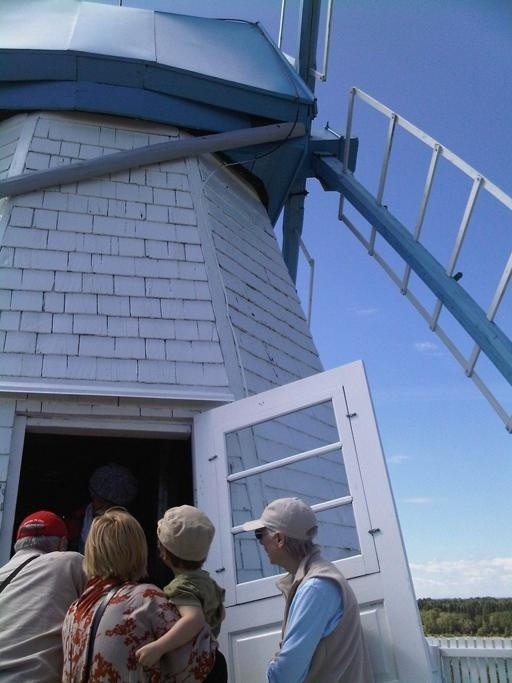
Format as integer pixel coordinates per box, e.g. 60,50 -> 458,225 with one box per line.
16,510 -> 71,542
240,495 -> 319,541
156,505 -> 216,562
87,463 -> 138,505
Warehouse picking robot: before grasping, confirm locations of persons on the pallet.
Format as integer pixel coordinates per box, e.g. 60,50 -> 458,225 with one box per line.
60,506 -> 217,683
236,498 -> 374,683
61,473 -> 124,555
134,506 -> 224,668
0,512 -> 88,683
